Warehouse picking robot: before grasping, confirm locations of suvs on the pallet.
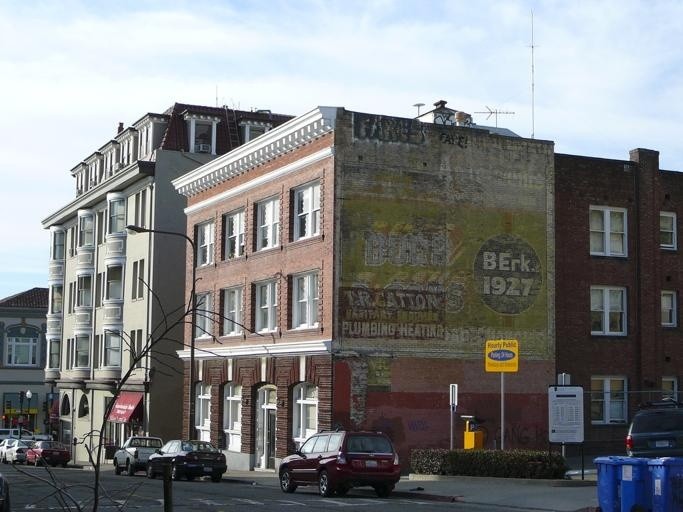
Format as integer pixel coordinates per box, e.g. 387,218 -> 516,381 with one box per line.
278,426 -> 401,499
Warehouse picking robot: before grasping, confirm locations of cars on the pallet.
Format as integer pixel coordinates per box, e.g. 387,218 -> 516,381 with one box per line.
0,438 -> 71,468
626,406 -> 683,459
113,436 -> 163,476
146,439 -> 228,483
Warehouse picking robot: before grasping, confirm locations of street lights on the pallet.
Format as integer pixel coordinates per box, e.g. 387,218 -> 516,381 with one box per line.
25,390 -> 33,431
122,224 -> 199,440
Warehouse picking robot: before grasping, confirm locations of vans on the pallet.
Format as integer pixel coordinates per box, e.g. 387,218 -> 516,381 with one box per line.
0,429 -> 34,438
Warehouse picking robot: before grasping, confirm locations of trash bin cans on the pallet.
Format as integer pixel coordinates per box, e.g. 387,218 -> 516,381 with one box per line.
594,455 -> 683,512
